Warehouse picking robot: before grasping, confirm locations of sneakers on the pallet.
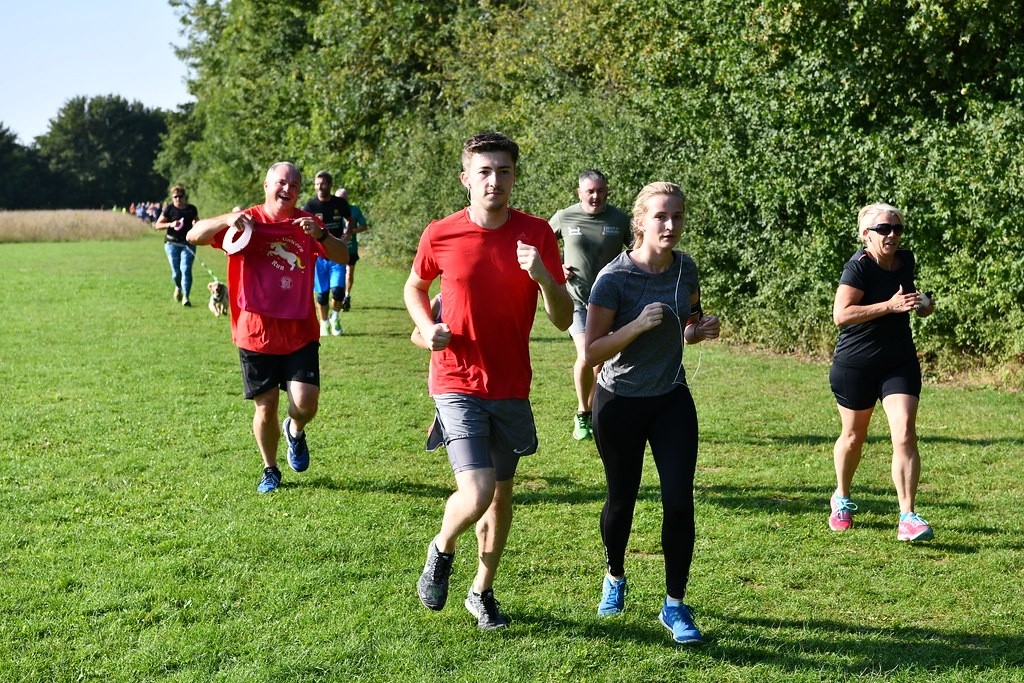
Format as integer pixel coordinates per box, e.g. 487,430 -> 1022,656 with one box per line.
257,462 -> 281,495
829,488 -> 858,533
897,512 -> 934,542
320,317 -> 329,337
464,585 -> 507,633
598,575 -> 629,620
573,407 -> 593,441
417,535 -> 455,611
282,416 -> 309,472
658,595 -> 702,644
329,318 -> 342,336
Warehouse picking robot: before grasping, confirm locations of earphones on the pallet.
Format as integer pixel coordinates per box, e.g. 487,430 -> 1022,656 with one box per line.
466,180 -> 470,186
867,234 -> 872,242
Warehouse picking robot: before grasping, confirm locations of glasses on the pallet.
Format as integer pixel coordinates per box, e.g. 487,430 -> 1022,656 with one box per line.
866,224 -> 905,235
173,195 -> 184,198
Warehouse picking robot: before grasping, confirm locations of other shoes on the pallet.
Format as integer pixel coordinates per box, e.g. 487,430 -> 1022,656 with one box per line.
341,298 -> 350,311
182,297 -> 191,306
174,286 -> 182,302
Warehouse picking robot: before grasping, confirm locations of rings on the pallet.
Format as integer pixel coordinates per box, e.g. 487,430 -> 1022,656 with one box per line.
307,226 -> 308,229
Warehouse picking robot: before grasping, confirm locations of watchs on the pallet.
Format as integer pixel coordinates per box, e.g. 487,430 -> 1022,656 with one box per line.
316,227 -> 328,242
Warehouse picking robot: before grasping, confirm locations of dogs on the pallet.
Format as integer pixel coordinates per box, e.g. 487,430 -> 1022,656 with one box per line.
207,281 -> 229,318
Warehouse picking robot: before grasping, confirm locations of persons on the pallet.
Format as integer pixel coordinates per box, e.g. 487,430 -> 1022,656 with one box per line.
121,206 -> 126,216
404,134 -> 575,629
130,201 -> 168,228
188,161 -> 350,492
304,172 -> 353,336
335,189 -> 368,312
548,170 -> 634,440
829,202 -> 935,543
112,204 -> 117,213
156,187 -> 200,306
585,182 -> 721,643
